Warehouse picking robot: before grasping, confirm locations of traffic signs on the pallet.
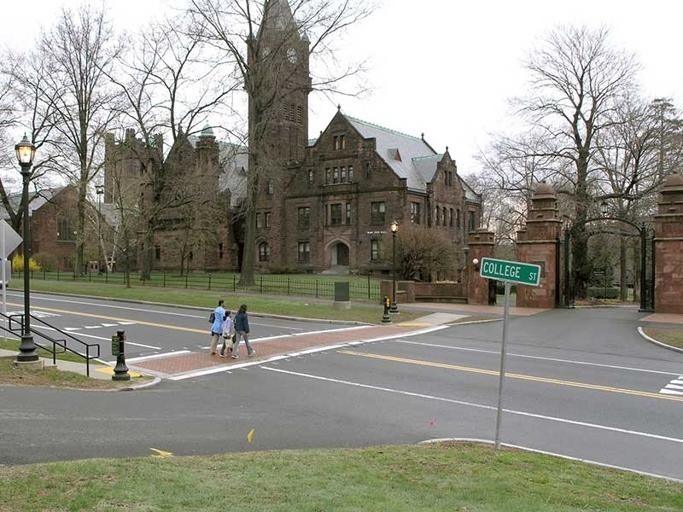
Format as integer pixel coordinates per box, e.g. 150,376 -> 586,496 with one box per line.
479,257 -> 541,288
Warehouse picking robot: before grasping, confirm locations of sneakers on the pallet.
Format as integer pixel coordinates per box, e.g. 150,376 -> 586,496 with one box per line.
212,350 -> 257,359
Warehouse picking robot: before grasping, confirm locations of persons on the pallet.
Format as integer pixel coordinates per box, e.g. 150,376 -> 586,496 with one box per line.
219,309 -> 235,357
229,304 -> 255,359
209,299 -> 227,355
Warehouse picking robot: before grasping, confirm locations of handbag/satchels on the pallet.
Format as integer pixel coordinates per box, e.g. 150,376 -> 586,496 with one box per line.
207,313 -> 215,323
225,339 -> 233,349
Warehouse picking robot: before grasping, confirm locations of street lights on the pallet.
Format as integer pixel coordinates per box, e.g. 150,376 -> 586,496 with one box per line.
388,219 -> 400,314
13,129 -> 39,362
93,185 -> 105,276
